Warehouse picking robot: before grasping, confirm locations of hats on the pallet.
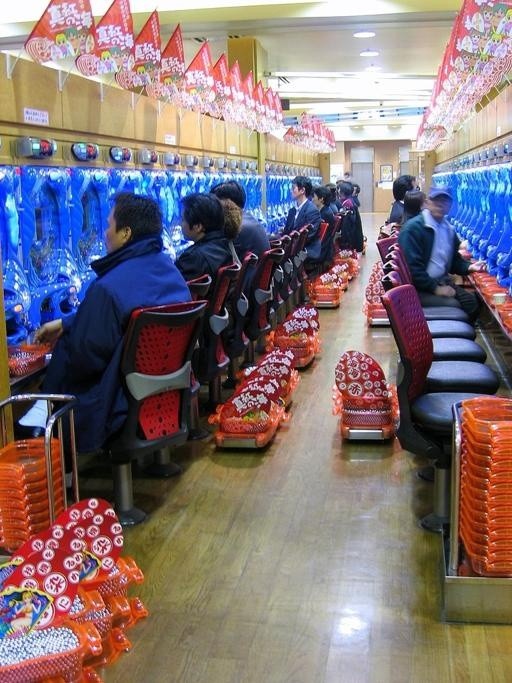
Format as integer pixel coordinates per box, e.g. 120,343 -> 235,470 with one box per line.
429,185 -> 454,200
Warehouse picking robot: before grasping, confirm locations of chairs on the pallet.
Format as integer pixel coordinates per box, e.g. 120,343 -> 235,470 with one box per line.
103,199 -> 360,529
376,218 -> 502,534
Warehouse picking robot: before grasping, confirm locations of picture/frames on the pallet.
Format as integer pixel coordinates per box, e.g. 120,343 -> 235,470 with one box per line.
379,163 -> 394,183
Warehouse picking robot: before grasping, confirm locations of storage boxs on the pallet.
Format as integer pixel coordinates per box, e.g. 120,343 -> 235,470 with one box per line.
1,439 -> 67,554
458,397 -> 512,578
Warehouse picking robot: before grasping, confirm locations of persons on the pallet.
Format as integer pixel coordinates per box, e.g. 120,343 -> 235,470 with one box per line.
215,198 -> 243,263
27,190 -> 196,500
170,194 -> 232,283
210,178 -> 274,262
277,169 -> 488,330
